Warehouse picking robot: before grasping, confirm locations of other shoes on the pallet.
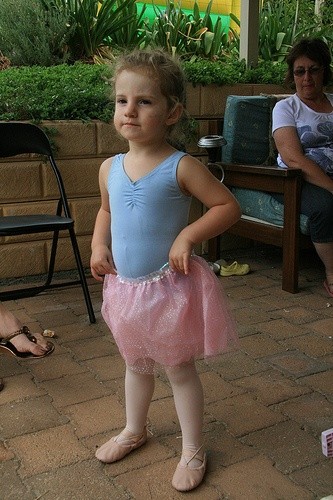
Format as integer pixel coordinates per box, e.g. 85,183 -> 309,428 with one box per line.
171,444 -> 207,493
94,427 -> 147,464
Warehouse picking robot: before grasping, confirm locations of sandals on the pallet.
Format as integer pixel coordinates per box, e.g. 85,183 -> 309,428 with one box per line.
218,258 -> 249,277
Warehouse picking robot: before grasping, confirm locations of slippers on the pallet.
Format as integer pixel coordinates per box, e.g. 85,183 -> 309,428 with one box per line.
1,322 -> 55,360
322,278 -> 333,297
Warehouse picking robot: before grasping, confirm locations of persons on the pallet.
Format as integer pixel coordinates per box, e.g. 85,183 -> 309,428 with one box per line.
272,38 -> 333,297
90,49 -> 242,491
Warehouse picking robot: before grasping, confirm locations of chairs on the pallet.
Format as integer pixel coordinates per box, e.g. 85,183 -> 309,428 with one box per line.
207,93 -> 310,295
0,122 -> 98,324
